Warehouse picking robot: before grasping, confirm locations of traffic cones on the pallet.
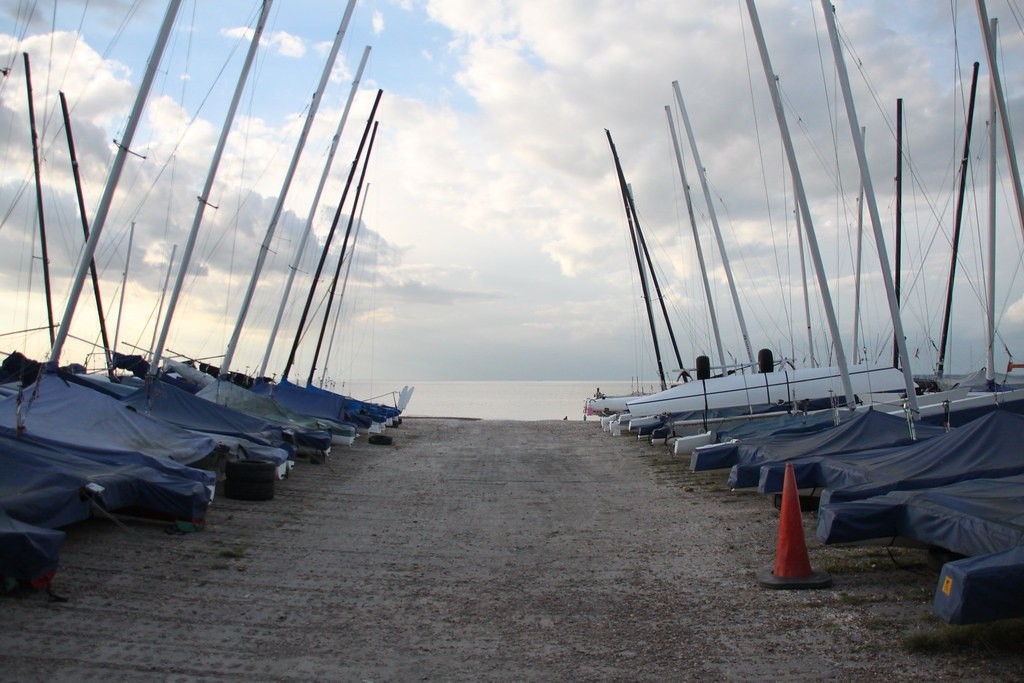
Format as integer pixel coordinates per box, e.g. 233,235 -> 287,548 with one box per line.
757,463 -> 832,588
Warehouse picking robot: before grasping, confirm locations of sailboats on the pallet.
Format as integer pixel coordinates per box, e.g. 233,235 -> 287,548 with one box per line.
585,0 -> 1023,626
0,0 -> 414,597
